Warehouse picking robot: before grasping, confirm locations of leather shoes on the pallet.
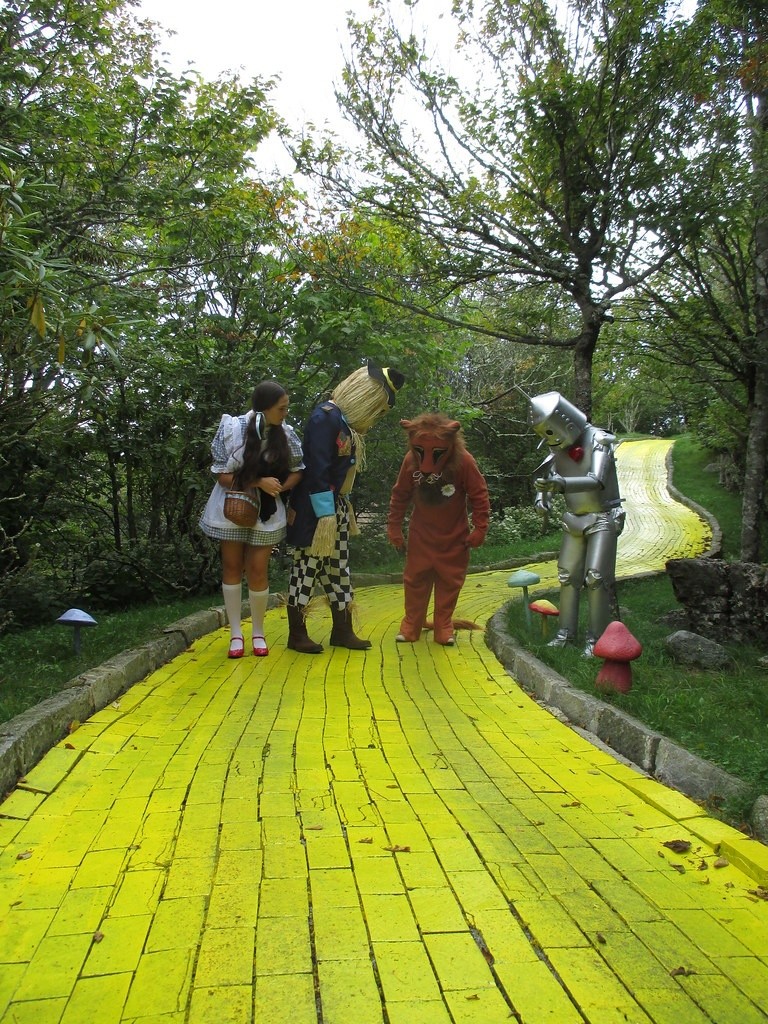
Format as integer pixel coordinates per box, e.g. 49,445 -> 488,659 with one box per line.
252,637 -> 268,655
229,634 -> 244,657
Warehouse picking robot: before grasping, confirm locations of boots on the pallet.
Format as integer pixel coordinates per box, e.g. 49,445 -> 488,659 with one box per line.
329,604 -> 371,650
287,603 -> 324,653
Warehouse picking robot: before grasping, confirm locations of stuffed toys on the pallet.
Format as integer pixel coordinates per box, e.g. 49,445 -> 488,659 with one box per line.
387,411 -> 490,646
285,359 -> 405,653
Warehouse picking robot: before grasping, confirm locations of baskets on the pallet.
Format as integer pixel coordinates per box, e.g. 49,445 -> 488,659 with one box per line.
223,475 -> 259,527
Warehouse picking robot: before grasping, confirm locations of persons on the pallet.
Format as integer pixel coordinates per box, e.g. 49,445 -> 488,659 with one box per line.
526,390 -> 627,659
197,380 -> 307,658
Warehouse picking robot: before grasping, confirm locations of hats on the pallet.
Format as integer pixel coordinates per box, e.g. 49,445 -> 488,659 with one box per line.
367,357 -> 405,408
